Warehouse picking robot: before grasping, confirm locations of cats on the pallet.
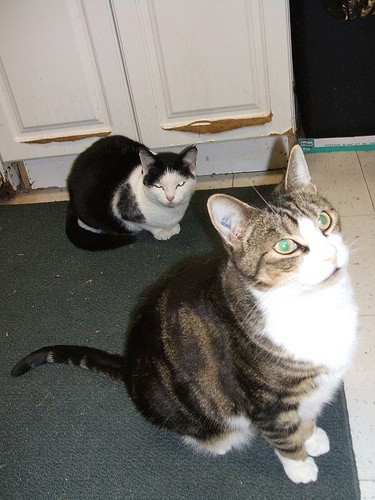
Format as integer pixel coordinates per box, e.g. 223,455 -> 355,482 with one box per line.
9,141 -> 361,486
64,134 -> 200,253
